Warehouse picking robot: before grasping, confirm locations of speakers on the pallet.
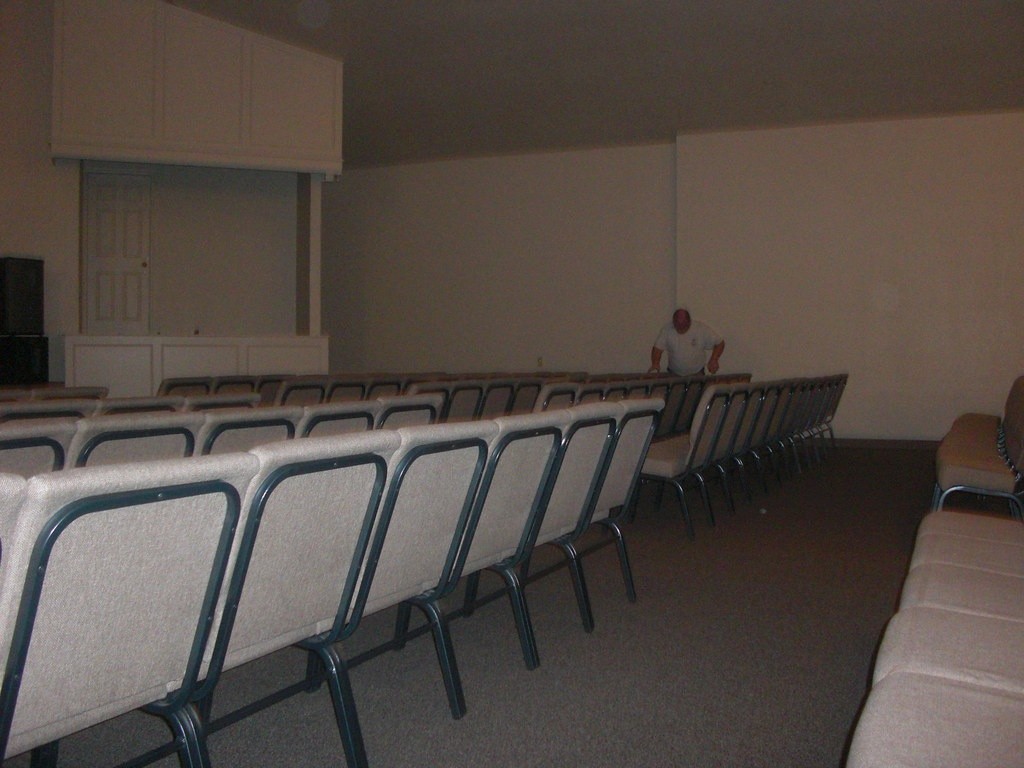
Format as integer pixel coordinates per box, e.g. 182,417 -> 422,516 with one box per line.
0,256 -> 45,336
0,336 -> 49,387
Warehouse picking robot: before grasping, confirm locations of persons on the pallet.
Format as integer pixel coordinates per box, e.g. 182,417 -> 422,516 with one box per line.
646,309 -> 726,376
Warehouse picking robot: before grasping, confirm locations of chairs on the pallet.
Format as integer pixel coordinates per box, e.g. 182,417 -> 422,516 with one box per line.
0,369 -> 1024,768
